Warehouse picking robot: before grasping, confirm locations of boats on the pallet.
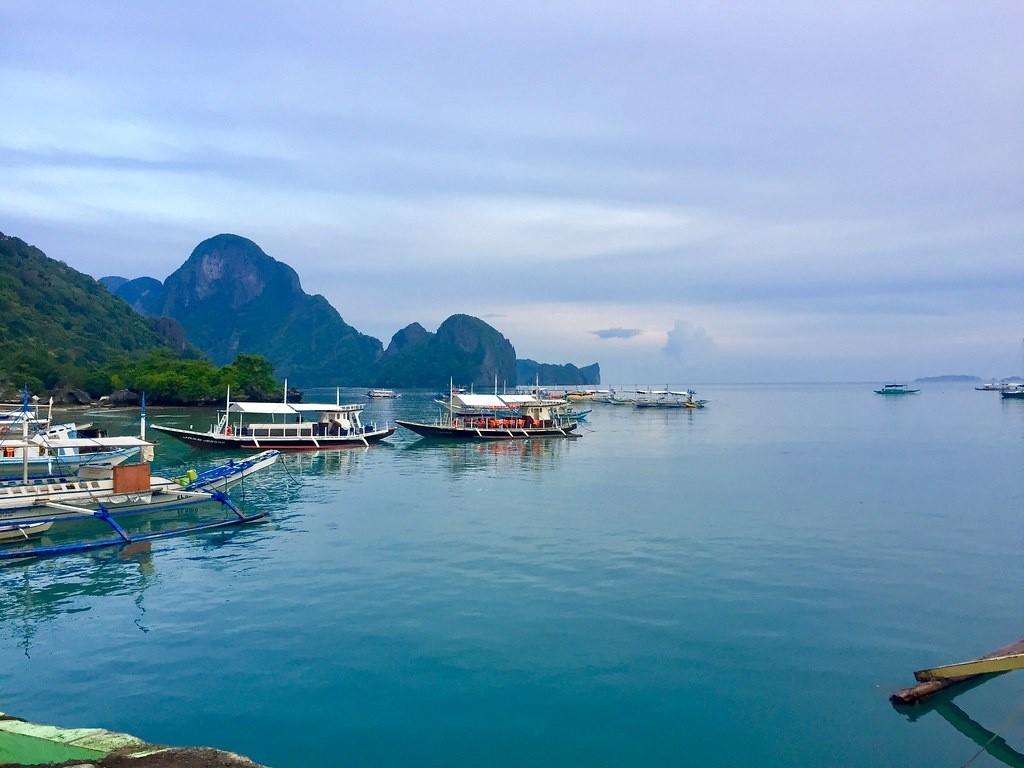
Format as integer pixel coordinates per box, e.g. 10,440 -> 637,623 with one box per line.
514,382 -> 710,409
439,383 -> 470,400
975,381 -> 1024,400
873,379 -> 920,394
0,381 -> 280,559
394,373 -> 582,438
549,406 -> 592,423
363,389 -> 401,399
150,378 -> 397,450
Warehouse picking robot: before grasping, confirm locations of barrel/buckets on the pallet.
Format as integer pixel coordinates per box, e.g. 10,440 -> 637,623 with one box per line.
187,470 -> 197,481
179,477 -> 189,486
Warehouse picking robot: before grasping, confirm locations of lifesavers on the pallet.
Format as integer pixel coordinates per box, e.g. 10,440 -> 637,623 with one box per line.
223,426 -> 232,436
452,419 -> 461,427
0,426 -> 11,436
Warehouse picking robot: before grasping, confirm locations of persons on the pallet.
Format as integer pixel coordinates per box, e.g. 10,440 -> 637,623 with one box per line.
330,419 -> 341,436
452,418 -> 460,427
466,417 -> 525,428
533,419 -> 539,427
224,425 -> 232,437
